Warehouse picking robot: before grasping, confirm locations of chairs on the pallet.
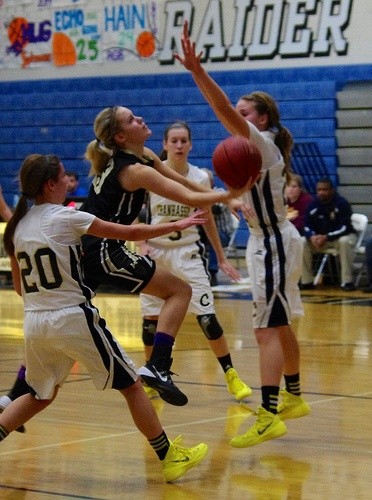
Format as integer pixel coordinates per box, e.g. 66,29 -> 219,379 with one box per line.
310,213 -> 368,289
208,215 -> 240,270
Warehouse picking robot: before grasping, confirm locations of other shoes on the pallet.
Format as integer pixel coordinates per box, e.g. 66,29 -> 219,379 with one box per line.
342,283 -> 356,290
299,283 -> 314,290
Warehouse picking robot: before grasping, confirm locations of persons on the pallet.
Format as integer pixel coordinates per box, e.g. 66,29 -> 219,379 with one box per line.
0,153 -> 208,483
173,39 -> 311,448
0,106 -> 261,432
132,122 -> 253,401
0,167 -> 358,293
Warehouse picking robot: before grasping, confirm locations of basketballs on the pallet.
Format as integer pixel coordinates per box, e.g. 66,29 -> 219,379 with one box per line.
212,137 -> 263,189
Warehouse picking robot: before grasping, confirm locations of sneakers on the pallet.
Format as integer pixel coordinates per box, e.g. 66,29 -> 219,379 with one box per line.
136,361 -> 189,407
228,403 -> 288,448
157,435 -> 208,482
224,368 -> 252,400
0,396 -> 25,433
276,389 -> 312,420
